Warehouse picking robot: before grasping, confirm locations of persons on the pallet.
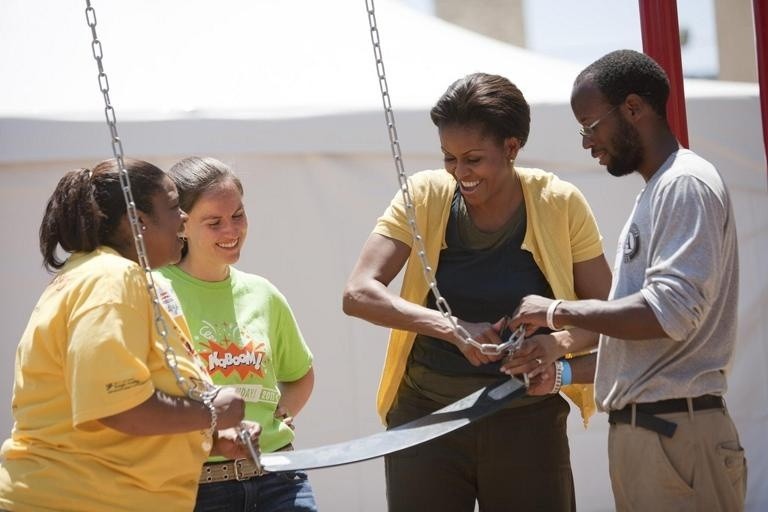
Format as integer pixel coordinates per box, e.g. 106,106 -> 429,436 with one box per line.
150,155 -> 319,512
0,156 -> 262,512
343,72 -> 611,512
509,50 -> 748,512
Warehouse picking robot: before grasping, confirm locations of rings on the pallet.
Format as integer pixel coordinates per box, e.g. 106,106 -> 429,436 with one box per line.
536,359 -> 542,365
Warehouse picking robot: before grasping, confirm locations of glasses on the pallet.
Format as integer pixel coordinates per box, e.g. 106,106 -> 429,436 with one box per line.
576,106 -> 618,139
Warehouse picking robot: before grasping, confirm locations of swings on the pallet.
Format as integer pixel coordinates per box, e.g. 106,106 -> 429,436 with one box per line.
84,1 -> 529,473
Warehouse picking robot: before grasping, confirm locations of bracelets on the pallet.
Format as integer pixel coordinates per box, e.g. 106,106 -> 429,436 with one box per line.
546,300 -> 562,332
202,398 -> 218,431
560,359 -> 572,386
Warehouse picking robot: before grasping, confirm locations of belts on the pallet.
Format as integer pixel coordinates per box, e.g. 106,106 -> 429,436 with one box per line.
608,395 -> 725,439
